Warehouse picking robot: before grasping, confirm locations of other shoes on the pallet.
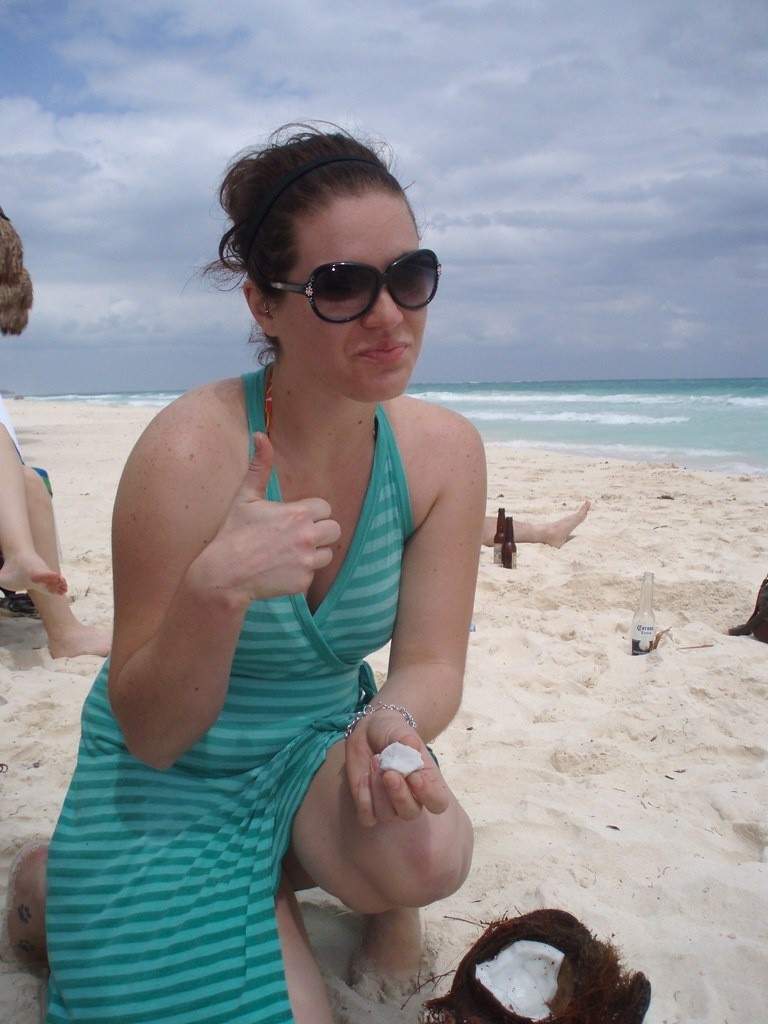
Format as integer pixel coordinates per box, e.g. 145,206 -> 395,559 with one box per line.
0,590 -> 41,619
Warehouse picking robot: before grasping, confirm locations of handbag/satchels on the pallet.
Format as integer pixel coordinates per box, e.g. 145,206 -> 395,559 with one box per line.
728,574 -> 768,643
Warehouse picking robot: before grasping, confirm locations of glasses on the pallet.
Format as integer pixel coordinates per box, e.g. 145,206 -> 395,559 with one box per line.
270,249 -> 442,324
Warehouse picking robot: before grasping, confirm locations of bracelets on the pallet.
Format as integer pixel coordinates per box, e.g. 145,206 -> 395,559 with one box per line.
344,700 -> 418,740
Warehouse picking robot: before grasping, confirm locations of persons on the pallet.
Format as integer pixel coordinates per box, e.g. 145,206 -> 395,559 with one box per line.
481,501 -> 591,549
6,135 -> 488,1024
0,393 -> 113,659
